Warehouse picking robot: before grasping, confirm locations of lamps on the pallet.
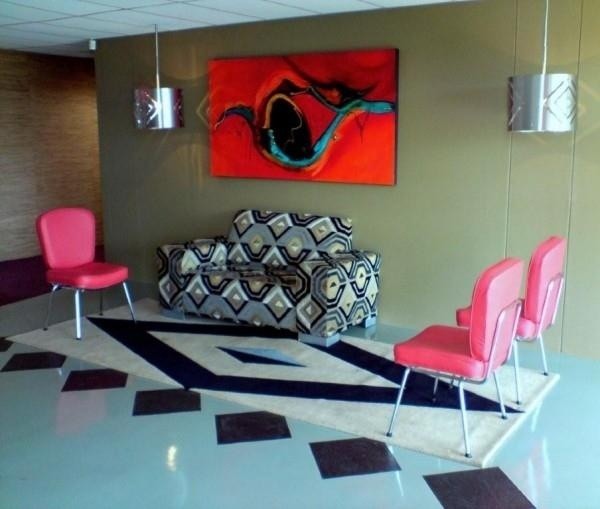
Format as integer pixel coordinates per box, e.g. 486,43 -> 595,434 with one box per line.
507,0 -> 576,133
130,29 -> 184,130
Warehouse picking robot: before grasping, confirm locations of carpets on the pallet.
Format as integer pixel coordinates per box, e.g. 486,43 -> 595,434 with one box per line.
8,297 -> 560,469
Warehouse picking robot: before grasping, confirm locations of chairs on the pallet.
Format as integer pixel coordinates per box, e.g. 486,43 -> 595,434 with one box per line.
387,258 -> 522,458
456,236 -> 566,403
35,208 -> 140,340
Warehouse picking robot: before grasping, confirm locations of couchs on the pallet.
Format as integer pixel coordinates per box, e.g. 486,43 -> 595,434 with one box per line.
154,209 -> 382,349
